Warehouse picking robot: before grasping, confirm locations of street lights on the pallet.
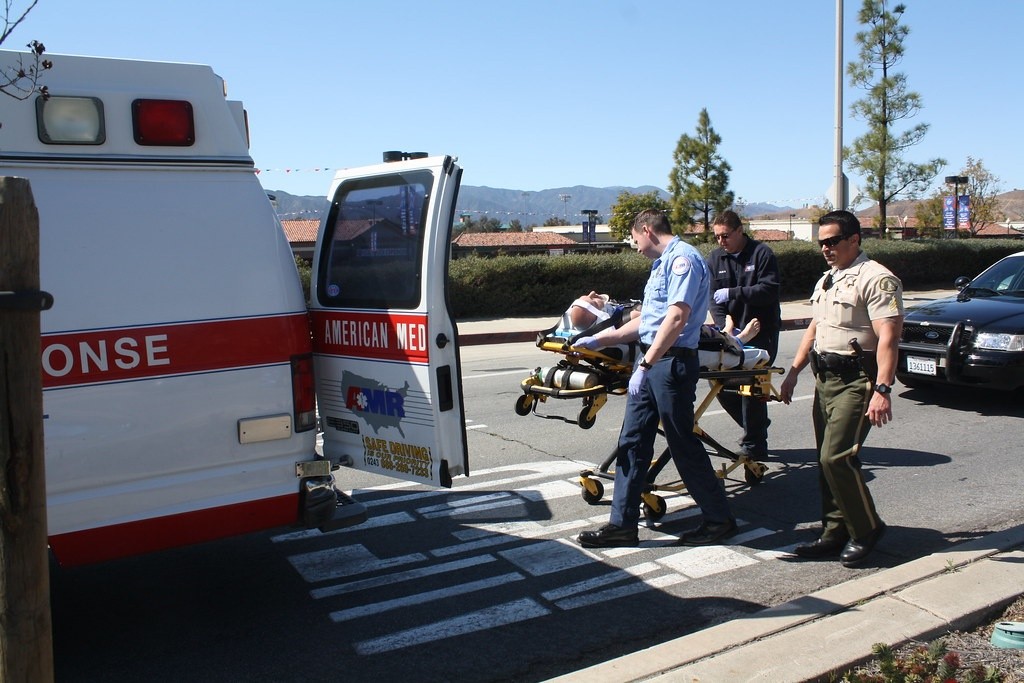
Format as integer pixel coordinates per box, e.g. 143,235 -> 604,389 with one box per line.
383,150 -> 429,261
943,176 -> 971,238
558,194 -> 573,221
581,210 -> 598,251
789,214 -> 796,241
904,216 -> 908,240
366,200 -> 382,261
1006,217 -> 1011,238
521,192 -> 530,227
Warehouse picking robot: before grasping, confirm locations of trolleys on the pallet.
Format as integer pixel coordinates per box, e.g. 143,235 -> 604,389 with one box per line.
514,292 -> 785,522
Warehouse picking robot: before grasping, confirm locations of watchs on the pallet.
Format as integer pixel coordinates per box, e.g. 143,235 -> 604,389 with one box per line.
638,357 -> 652,370
874,383 -> 891,394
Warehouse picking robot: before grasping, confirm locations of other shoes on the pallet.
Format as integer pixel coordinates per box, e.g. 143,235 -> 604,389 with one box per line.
738,418 -> 771,447
732,450 -> 767,462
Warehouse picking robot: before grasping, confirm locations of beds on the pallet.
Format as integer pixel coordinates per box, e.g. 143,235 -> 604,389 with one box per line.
541,338 -> 785,379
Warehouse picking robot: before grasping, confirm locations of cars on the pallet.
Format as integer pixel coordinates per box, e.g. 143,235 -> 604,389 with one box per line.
895,250 -> 1024,404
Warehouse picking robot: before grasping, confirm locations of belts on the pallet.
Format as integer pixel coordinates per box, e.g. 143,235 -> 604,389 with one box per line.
645,343 -> 698,358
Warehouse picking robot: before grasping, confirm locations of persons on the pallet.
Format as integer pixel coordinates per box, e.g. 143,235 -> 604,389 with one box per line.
706,211 -> 787,462
571,291 -> 760,350
572,207 -> 737,548
780,211 -> 903,564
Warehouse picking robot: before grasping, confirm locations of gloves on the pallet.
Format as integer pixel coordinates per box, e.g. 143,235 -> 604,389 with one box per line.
572,337 -> 599,357
733,328 -> 741,336
714,287 -> 731,304
628,369 -> 646,396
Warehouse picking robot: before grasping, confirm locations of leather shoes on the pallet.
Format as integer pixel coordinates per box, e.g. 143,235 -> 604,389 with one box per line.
795,535 -> 845,557
576,523 -> 638,547
681,516 -> 739,547
840,521 -> 886,566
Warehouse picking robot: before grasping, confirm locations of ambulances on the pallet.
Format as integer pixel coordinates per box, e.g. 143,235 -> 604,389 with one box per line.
0,49 -> 471,570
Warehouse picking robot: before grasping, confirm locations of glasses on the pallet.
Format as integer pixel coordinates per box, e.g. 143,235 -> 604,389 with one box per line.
714,226 -> 739,241
817,230 -> 856,248
